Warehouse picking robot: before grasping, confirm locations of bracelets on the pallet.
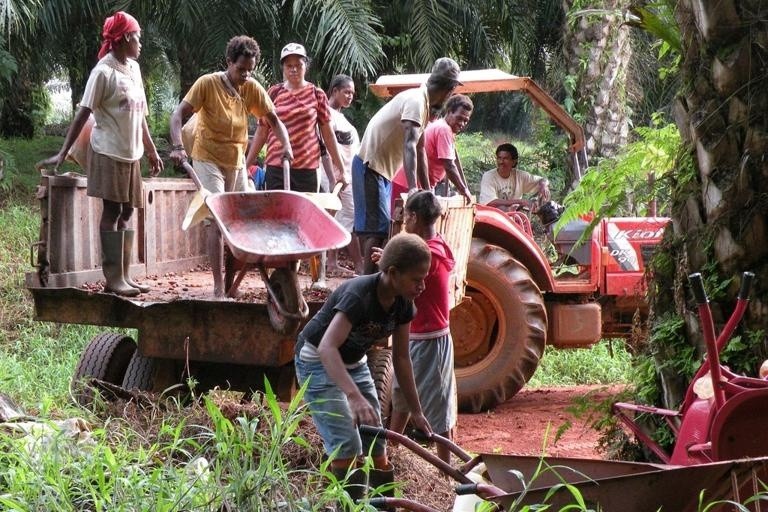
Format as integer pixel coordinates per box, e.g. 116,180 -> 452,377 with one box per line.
170,142 -> 183,151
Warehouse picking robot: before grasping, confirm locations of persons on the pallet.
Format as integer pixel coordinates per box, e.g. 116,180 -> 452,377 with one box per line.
245,141 -> 266,190
390,93 -> 475,229
243,41 -> 349,195
368,189 -> 461,481
348,56 -> 466,276
313,75 -> 364,276
166,34 -> 295,300
35,10 -> 163,299
294,231 -> 435,511
35,107 -> 95,173
478,143 -> 575,264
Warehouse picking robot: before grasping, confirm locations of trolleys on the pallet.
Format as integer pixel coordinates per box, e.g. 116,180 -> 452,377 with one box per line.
615,269 -> 767,511
353,422 -> 767,512
176,157 -> 353,337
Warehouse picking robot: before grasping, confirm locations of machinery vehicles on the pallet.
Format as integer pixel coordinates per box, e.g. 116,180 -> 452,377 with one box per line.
25,66 -> 683,424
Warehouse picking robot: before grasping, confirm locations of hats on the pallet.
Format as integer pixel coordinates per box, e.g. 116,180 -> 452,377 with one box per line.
279,42 -> 309,61
432,57 -> 465,87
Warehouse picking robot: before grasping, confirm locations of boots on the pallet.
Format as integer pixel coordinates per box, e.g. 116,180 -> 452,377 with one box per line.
100,228 -> 151,296
331,460 -> 394,512
